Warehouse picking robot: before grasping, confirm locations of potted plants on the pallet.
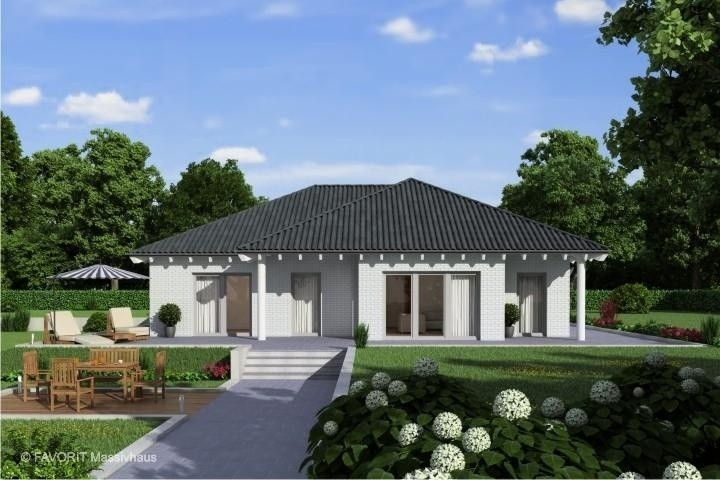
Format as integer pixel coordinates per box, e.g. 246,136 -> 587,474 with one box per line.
158,303 -> 182,337
505,304 -> 521,338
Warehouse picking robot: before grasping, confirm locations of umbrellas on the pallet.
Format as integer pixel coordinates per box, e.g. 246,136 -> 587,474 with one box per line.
46,259 -> 149,290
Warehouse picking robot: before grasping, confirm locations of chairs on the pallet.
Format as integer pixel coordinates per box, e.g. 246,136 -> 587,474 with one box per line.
43,310 -> 114,346
23,348 -> 165,413
106,306 -> 150,342
